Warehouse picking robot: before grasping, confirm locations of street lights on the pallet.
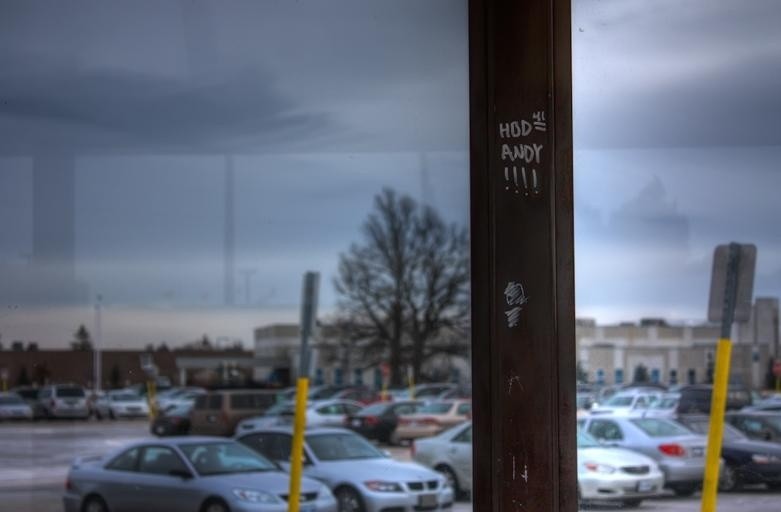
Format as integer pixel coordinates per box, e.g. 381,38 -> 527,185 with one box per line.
89,292 -> 109,396
